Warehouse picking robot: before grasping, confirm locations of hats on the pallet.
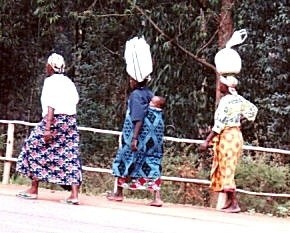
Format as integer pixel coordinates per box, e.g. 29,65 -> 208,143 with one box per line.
220,74 -> 238,87
47,53 -> 65,72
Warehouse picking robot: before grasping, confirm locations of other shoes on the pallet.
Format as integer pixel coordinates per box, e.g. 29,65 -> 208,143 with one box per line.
61,199 -> 79,205
15,192 -> 37,199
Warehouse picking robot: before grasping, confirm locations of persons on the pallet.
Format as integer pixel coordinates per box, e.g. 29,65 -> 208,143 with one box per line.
143,96 -> 164,153
202,75 -> 258,213
107,76 -> 163,206
16,53 -> 82,204
